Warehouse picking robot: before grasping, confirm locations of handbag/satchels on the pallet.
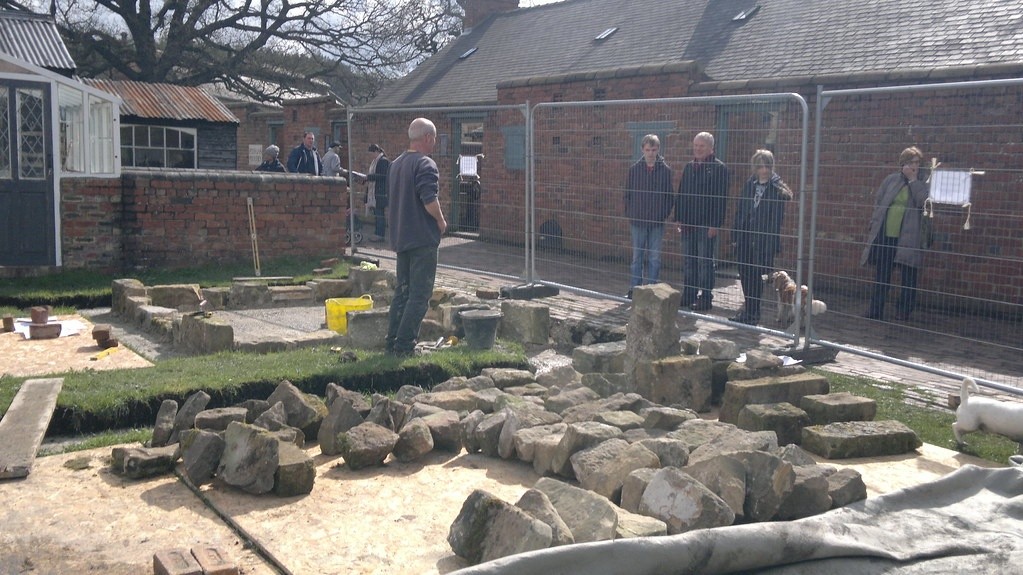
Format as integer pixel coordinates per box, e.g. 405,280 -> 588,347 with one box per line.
921,225 -> 928,248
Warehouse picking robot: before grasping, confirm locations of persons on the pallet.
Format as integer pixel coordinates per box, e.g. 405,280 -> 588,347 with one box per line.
621,133 -> 675,299
729,150 -> 792,325
361,144 -> 390,242
385,118 -> 447,355
675,132 -> 729,310
255,144 -> 287,173
288,131 -> 323,176
321,140 -> 349,177
860,146 -> 932,322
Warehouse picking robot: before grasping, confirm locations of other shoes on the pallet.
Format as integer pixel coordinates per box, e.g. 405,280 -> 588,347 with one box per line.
679,298 -> 696,306
896,307 -> 909,321
691,296 -> 712,310
727,310 -> 761,325
623,289 -> 632,300
367,234 -> 385,242
385,346 -> 423,356
857,310 -> 879,322
393,350 -> 432,358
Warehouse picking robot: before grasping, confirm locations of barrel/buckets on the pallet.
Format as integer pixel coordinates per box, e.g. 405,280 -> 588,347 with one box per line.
325,294 -> 374,335
458,310 -> 504,349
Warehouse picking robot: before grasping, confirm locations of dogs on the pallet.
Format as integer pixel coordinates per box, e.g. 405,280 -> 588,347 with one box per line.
766,270 -> 828,326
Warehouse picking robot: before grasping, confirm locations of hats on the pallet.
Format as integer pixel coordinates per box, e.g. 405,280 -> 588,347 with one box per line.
265,144 -> 280,158
330,141 -> 343,149
